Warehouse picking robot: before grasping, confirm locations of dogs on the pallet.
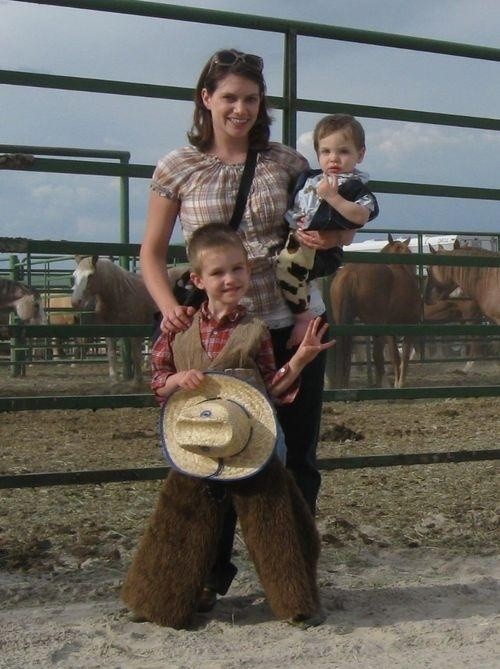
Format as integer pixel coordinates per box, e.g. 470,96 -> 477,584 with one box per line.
70,252 -> 192,394
326,232 -> 424,389
422,242 -> 500,328
414,275 -> 482,376
40,288 -> 90,369
0,277 -> 49,326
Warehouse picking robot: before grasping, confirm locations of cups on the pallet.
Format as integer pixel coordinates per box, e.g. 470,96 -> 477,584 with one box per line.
206,51 -> 263,84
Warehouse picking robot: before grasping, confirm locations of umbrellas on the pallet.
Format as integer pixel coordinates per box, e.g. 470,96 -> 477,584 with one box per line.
195,589 -> 216,612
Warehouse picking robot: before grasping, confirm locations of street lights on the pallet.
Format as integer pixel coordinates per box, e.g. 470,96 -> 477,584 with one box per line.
148,270 -> 208,349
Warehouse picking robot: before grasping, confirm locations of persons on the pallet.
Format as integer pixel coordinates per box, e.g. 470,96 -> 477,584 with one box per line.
275,113 -> 379,347
118,224 -> 339,630
140,50 -> 356,613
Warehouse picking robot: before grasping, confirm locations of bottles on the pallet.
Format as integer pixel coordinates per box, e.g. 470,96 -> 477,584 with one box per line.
160,372 -> 278,481
